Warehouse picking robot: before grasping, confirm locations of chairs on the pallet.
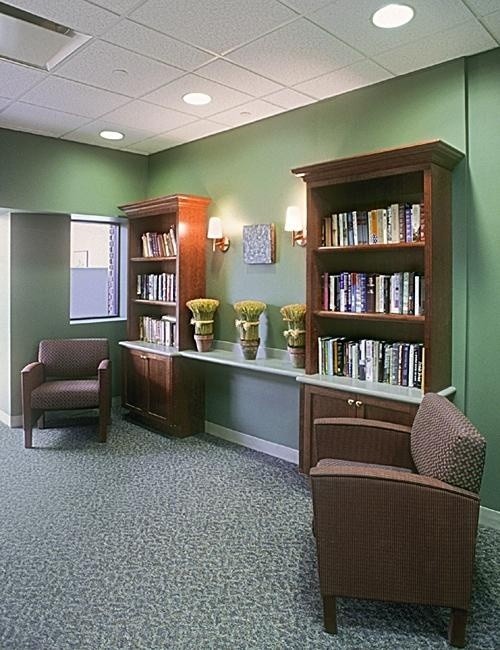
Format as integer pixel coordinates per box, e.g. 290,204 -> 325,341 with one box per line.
309,390 -> 488,648
21,337 -> 112,448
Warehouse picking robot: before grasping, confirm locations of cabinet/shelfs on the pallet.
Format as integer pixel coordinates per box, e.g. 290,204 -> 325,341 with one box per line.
116,192 -> 213,438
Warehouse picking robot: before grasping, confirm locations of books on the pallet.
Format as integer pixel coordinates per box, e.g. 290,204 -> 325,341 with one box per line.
135,227 -> 177,346
317,201 -> 425,388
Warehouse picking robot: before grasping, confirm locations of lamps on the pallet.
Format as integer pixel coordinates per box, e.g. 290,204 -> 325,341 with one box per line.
207,217 -> 230,253
284,206 -> 307,247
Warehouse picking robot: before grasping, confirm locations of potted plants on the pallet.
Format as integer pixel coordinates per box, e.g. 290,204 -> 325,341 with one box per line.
185,298 -> 306,368
